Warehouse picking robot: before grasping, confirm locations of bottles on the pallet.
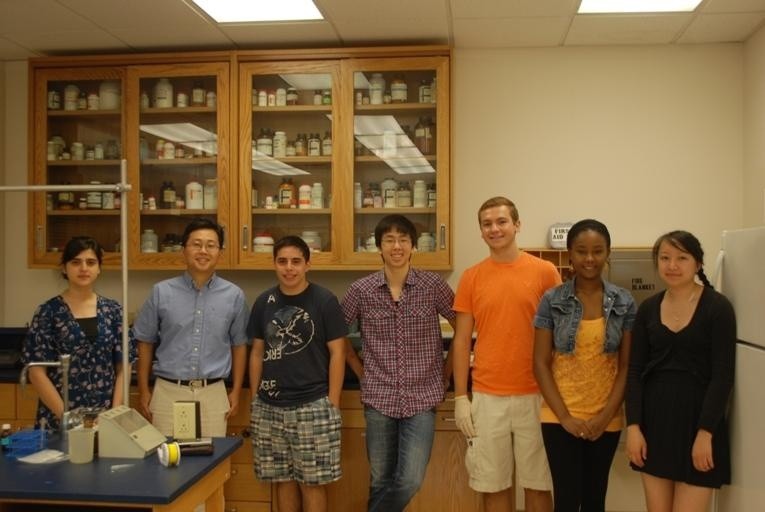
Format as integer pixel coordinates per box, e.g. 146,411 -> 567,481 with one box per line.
353,177 -> 436,208
354,138 -> 374,155
370,232 -> 376,252
300,232 -> 321,252
47,80 -> 122,110
418,78 -> 436,104
313,89 -> 331,105
47,136 -> 121,160
140,78 -> 216,108
399,116 -> 436,155
139,178 -> 217,210
252,128 -> 332,156
252,176 -> 324,209
140,229 -> 183,252
46,181 -> 121,210
48,240 -> 59,252
139,136 -> 202,160
356,73 -> 408,105
252,87 -> 298,107
417,232 -> 434,252
252,233 -> 275,252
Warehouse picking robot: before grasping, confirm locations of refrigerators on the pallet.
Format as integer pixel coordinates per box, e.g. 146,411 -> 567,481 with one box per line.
711,226 -> 765,512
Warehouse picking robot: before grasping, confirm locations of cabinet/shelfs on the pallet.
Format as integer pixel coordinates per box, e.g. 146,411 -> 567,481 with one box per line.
522,248 -> 577,286
27,51 -> 239,272
272,392 -> 484,512
239,43 -> 455,273
1,384 -> 272,512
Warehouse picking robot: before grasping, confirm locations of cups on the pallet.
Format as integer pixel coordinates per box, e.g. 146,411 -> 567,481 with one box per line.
68,428 -> 95,465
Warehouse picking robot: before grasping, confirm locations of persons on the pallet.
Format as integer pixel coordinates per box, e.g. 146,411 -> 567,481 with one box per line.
624,231 -> 736,512
131,218 -> 252,512
245,235 -> 349,512
450,197 -> 563,512
20,236 -> 138,438
339,213 -> 457,512
532,219 -> 638,512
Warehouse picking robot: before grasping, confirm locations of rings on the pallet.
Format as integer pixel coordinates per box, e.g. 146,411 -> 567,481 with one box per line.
581,432 -> 584,437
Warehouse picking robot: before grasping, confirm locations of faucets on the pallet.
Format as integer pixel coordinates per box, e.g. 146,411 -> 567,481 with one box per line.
20,354 -> 72,432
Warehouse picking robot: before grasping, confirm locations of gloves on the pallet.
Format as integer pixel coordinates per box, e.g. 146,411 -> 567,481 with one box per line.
454,394 -> 476,438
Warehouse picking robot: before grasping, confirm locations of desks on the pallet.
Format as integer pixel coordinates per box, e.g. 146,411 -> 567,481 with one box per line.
0,425 -> 244,512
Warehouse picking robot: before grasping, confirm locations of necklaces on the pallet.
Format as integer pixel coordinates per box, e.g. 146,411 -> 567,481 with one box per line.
669,291 -> 695,321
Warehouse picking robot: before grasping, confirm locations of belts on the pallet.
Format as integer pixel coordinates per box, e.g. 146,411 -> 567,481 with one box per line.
157,375 -> 223,389
